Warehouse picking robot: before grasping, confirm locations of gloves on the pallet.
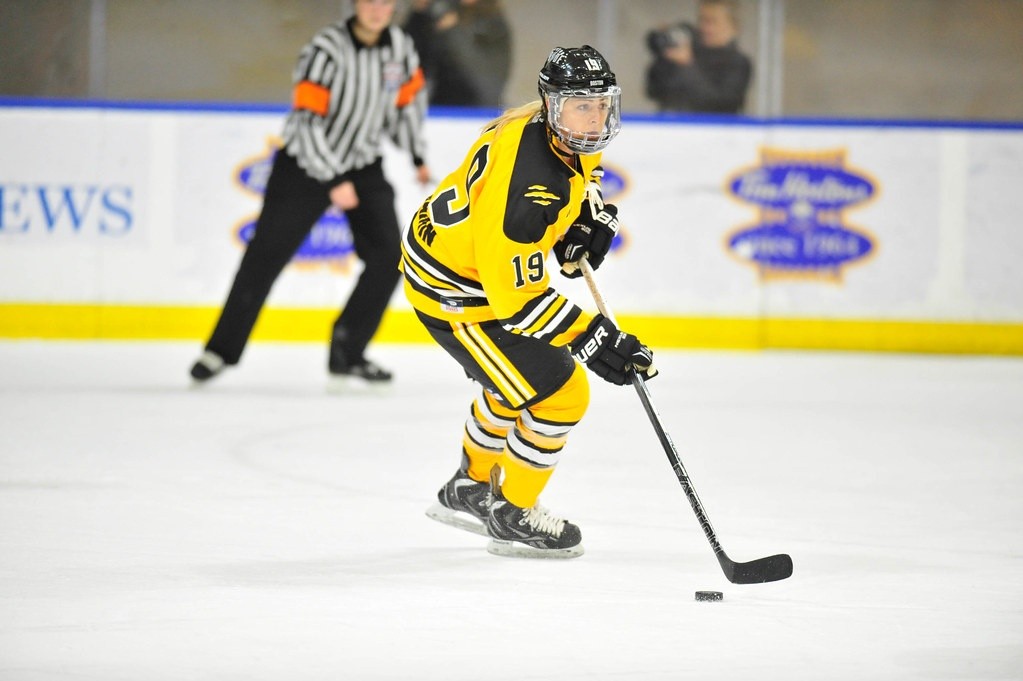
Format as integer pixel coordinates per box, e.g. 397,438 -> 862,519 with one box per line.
568,313 -> 659,385
552,203 -> 620,279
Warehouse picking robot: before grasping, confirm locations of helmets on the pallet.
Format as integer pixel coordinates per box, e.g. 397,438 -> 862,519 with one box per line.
538,44 -> 622,152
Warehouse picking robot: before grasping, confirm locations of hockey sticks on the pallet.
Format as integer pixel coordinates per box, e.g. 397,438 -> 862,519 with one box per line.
579,253 -> 793,585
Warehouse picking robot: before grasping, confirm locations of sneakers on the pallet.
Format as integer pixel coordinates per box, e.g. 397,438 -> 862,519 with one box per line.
486,463 -> 584,558
424,446 -> 509,536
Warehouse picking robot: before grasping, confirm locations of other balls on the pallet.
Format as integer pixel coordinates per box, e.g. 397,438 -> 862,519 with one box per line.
695,591 -> 724,603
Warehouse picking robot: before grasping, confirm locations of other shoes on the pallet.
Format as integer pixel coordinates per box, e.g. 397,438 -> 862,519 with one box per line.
184,349 -> 227,386
325,352 -> 392,395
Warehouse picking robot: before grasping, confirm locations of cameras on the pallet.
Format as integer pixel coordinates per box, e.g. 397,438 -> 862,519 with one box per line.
647,22 -> 701,54
425,0 -> 452,21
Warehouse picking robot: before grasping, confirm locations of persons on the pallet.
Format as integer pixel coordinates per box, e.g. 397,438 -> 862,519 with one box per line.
641,0 -> 750,116
396,45 -> 659,556
182,0 -> 436,385
402,0 -> 513,109
